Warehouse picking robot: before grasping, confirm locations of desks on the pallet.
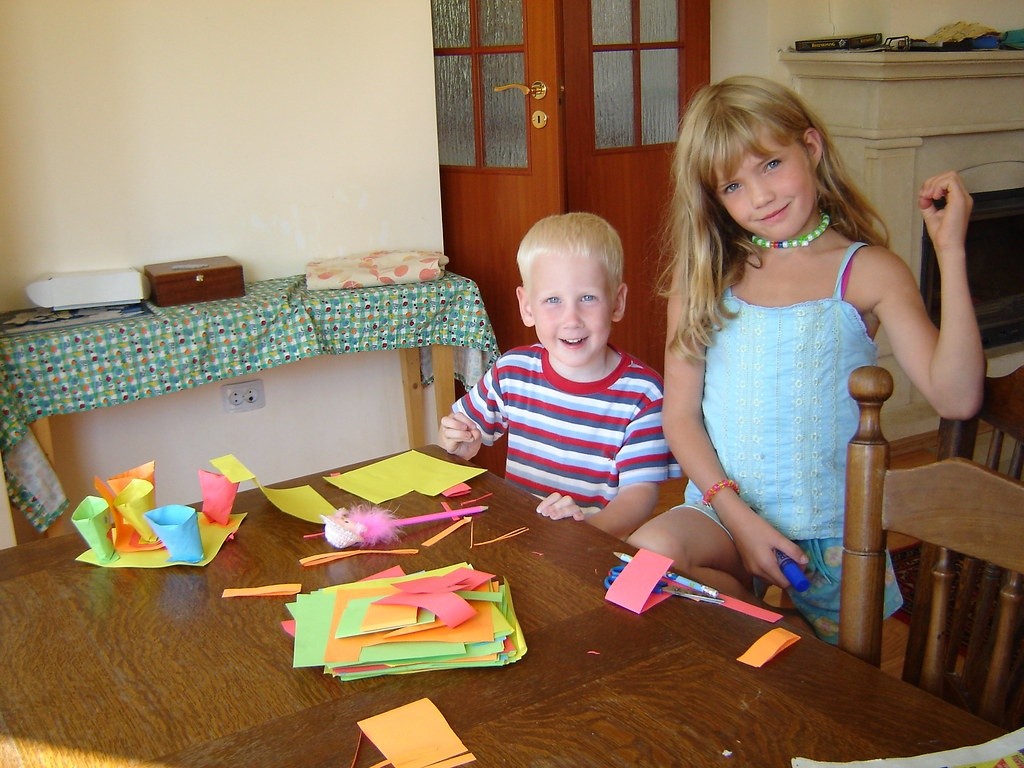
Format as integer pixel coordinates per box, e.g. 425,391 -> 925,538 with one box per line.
0,270 -> 504,542
0,446 -> 1023,768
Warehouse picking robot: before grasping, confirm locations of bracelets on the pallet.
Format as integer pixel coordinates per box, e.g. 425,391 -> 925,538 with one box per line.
703,479 -> 740,505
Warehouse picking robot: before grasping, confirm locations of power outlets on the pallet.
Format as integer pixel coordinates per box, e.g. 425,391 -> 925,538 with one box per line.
221,379 -> 266,414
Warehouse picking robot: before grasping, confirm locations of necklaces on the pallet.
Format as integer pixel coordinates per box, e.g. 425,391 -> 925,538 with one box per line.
752,207 -> 829,248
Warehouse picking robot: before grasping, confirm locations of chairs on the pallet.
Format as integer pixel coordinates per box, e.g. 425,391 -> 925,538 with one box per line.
841,365 -> 1023,733
901,364 -> 1024,727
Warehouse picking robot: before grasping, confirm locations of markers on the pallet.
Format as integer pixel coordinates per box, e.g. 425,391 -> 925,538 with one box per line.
770,546 -> 810,594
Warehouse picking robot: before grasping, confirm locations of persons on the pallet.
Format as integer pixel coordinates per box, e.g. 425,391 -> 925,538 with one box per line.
625,77 -> 986,649
438,212 -> 687,543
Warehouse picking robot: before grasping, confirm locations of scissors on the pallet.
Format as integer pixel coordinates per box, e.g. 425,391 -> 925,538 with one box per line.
604,564 -> 724,605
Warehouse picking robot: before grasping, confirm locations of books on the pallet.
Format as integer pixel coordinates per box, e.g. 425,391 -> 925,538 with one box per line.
0,302 -> 155,339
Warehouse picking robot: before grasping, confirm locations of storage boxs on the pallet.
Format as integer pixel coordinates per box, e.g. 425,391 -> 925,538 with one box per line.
144,255 -> 246,306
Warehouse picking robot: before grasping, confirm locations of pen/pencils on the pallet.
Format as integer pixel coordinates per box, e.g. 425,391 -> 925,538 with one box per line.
611,549 -> 719,598
322,503 -> 490,550
